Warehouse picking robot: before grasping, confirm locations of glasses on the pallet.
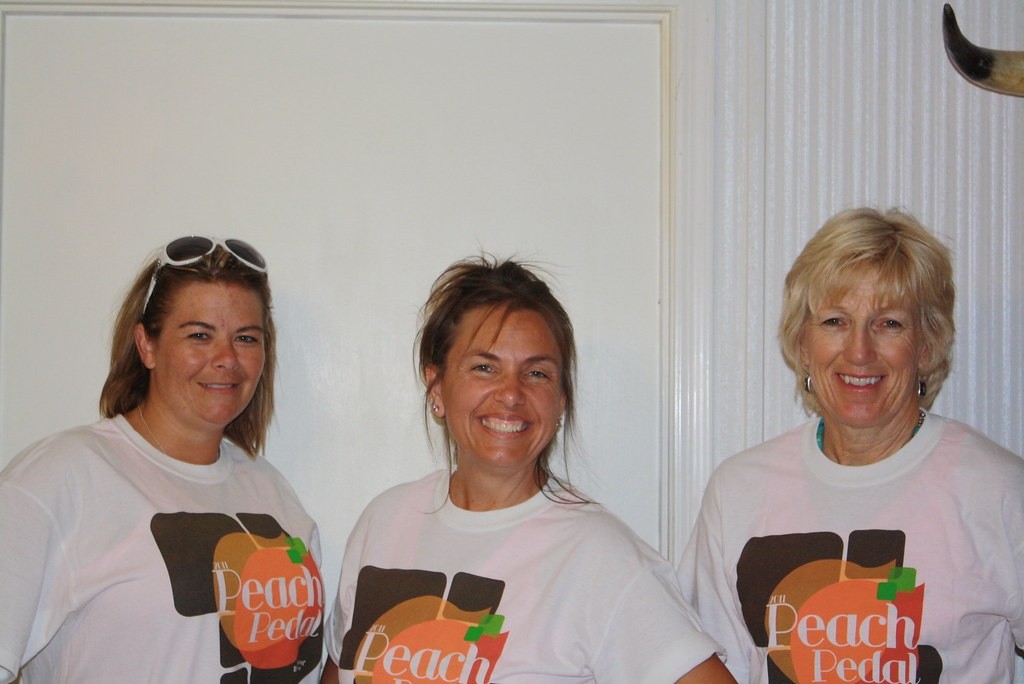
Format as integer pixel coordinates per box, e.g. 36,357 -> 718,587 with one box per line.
140,233 -> 268,315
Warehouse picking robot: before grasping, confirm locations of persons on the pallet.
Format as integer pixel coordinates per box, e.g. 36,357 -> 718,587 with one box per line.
318,257 -> 738,684
676,208 -> 1022,684
1,236 -> 325,684
